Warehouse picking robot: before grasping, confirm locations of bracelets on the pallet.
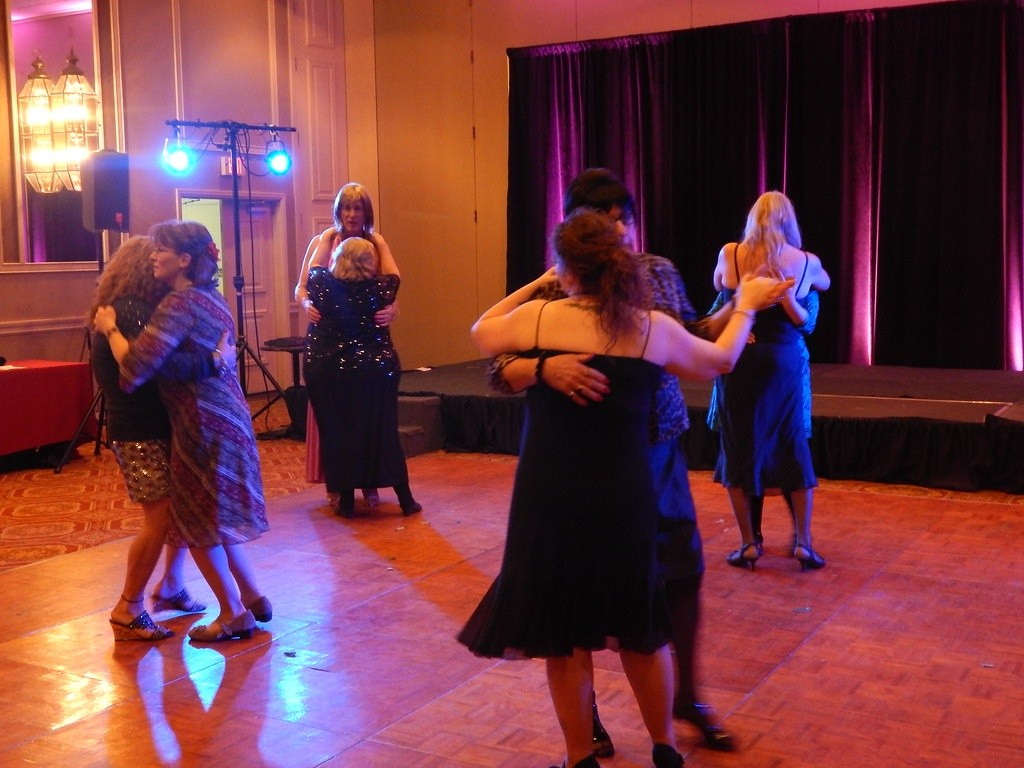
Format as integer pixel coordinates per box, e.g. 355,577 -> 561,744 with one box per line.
302,299 -> 313,308
391,304 -> 400,322
732,308 -> 755,320
214,350 -> 228,379
534,351 -> 547,384
107,326 -> 119,342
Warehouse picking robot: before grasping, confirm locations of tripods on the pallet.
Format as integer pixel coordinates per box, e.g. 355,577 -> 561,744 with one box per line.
54,231 -> 110,474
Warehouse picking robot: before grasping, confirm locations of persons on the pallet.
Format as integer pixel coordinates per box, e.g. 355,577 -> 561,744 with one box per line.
294,182 -> 424,517
92,219 -> 272,641
710,190 -> 832,574
85,235 -> 238,641
457,172 -> 797,768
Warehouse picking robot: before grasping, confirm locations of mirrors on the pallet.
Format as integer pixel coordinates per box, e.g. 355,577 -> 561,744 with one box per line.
4,1 -> 108,263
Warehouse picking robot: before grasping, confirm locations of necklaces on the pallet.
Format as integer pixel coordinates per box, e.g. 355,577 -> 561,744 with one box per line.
181,281 -> 192,292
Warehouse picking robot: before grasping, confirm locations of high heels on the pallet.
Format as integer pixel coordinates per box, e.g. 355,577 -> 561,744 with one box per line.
794,544 -> 825,572
727,543 -> 760,572
109,610 -> 175,641
152,586 -> 207,613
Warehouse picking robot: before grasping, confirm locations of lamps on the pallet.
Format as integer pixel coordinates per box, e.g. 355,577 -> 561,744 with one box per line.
16,52 -> 64,192
51,42 -> 97,192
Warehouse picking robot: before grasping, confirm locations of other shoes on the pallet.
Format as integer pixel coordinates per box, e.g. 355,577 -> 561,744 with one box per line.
403,502 -> 422,515
335,503 -> 353,517
673,688 -> 731,749
326,488 -> 340,508
247,596 -> 272,622
591,704 -> 614,757
652,744 -> 684,768
188,609 -> 256,641
362,488 -> 379,508
549,754 -> 600,768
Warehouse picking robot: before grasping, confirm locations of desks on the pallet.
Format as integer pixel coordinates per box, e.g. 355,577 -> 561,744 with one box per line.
0,357 -> 99,454
261,337 -> 309,433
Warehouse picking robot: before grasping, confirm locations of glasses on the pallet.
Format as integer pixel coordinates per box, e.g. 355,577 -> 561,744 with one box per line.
614,210 -> 637,226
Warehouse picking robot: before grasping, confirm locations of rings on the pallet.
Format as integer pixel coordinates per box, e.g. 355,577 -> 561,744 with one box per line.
231,346 -> 237,350
585,368 -> 590,375
778,296 -> 785,300
578,385 -> 584,392
568,391 -> 574,397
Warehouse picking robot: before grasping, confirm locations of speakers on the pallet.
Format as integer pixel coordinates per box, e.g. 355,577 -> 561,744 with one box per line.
80,152 -> 128,232
